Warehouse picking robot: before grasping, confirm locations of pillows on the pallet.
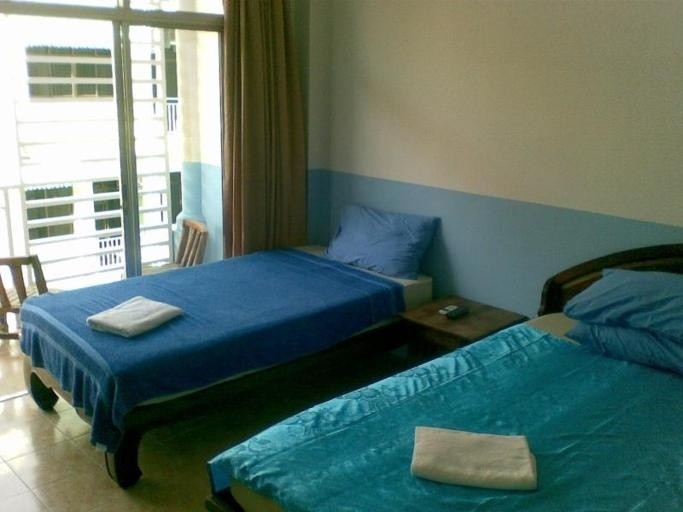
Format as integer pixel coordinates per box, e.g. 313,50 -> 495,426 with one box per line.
564,267 -> 683,375
321,204 -> 440,281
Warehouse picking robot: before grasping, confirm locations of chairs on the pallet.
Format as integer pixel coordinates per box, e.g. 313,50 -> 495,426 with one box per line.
0,218 -> 208,340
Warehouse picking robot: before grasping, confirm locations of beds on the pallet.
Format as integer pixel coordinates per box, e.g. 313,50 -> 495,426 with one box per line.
20,240 -> 434,492
205,241 -> 683,512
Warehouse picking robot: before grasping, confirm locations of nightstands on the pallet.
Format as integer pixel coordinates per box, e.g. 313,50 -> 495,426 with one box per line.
399,294 -> 527,351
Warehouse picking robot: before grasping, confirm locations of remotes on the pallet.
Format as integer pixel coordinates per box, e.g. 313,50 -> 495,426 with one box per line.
446,306 -> 470,319
438,304 -> 458,316
